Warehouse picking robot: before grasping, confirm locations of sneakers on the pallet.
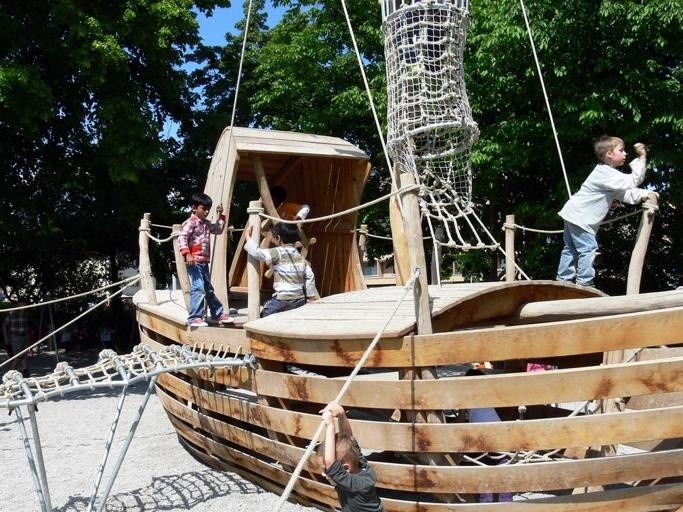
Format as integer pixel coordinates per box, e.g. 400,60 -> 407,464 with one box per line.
212,313 -> 234,322
187,317 -> 208,326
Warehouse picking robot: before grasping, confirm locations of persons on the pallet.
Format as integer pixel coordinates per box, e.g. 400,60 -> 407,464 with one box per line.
60,332 -> 72,354
556,134 -> 660,287
1,296 -> 31,378
318,401 -> 387,512
178,192 -> 235,327
270,185 -> 310,220
458,368 -> 513,502
243,221 -> 320,318
